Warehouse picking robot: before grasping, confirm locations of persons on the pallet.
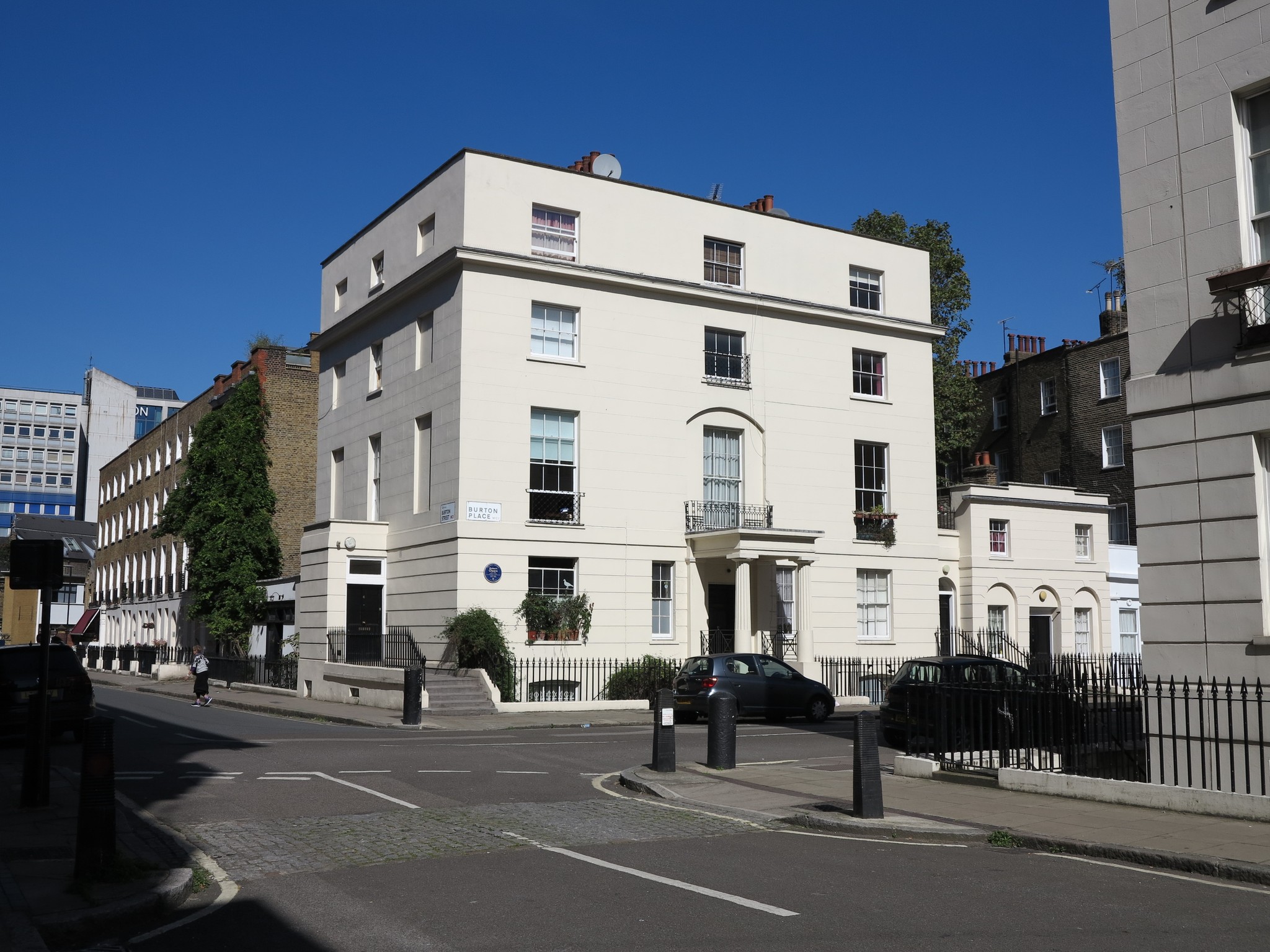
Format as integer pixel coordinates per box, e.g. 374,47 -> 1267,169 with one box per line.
184,645 -> 212,707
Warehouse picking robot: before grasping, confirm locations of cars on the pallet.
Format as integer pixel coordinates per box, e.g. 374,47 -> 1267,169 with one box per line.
669,652 -> 836,722
877,654 -> 1071,749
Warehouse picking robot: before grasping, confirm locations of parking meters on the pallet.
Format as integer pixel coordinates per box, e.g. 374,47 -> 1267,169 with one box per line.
8,537 -> 66,811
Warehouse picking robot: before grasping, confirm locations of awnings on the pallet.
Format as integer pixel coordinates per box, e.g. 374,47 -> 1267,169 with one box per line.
69,607 -> 101,635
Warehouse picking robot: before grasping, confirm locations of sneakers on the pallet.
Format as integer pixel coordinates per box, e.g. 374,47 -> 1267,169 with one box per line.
203,697 -> 212,706
191,702 -> 201,707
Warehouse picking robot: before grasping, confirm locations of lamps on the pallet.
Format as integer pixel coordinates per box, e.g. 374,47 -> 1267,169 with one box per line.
270,592 -> 284,601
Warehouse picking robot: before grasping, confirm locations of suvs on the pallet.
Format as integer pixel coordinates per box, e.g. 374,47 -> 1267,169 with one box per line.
0,642 -> 95,748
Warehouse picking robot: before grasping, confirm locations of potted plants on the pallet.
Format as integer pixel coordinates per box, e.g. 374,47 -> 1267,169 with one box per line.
513,590 -> 594,646
1205,260 -> 1270,295
853,505 -> 899,519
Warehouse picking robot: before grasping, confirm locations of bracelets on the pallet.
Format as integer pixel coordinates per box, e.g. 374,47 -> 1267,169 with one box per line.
187,674 -> 189,676
